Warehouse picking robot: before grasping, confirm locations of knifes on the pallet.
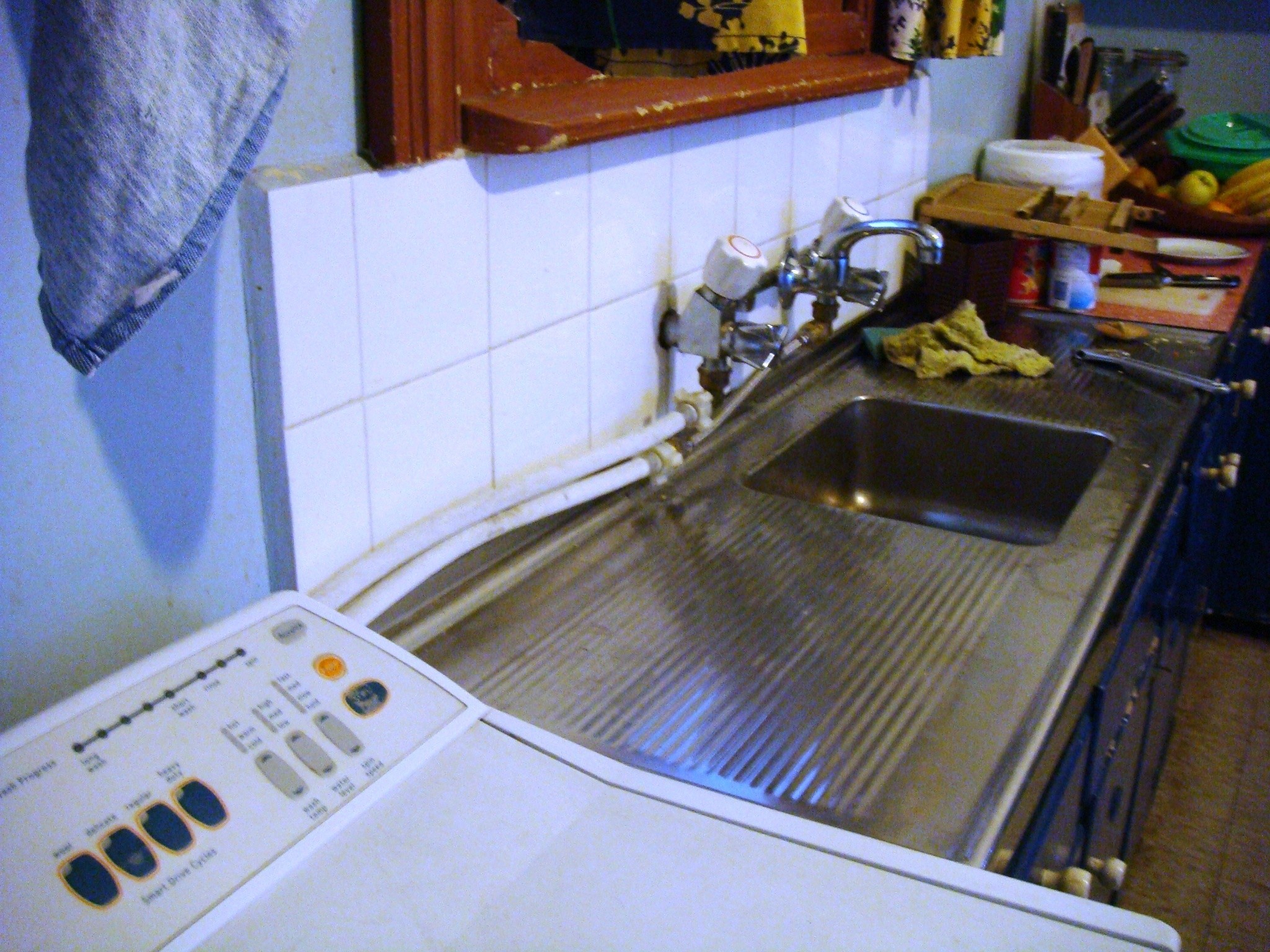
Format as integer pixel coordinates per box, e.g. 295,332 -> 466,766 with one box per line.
1038,3 -> 1098,110
1099,271 -> 1241,289
1098,71 -> 1185,160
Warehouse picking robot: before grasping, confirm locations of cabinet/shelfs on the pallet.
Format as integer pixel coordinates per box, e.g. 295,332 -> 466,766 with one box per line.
988,242 -> 1270,914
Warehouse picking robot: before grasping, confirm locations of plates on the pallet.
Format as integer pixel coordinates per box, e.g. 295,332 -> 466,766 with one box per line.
1153,238 -> 1252,264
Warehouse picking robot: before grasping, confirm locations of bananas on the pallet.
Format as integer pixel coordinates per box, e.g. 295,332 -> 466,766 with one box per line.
1218,158 -> 1270,217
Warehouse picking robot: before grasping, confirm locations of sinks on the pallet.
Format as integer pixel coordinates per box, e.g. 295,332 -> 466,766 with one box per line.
741,389 -> 1120,550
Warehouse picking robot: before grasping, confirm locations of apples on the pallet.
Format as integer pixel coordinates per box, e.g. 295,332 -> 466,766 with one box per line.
1127,168 -> 1218,204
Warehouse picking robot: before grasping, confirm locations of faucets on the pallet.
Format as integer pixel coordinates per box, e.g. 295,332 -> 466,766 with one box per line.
781,194 -> 945,312
